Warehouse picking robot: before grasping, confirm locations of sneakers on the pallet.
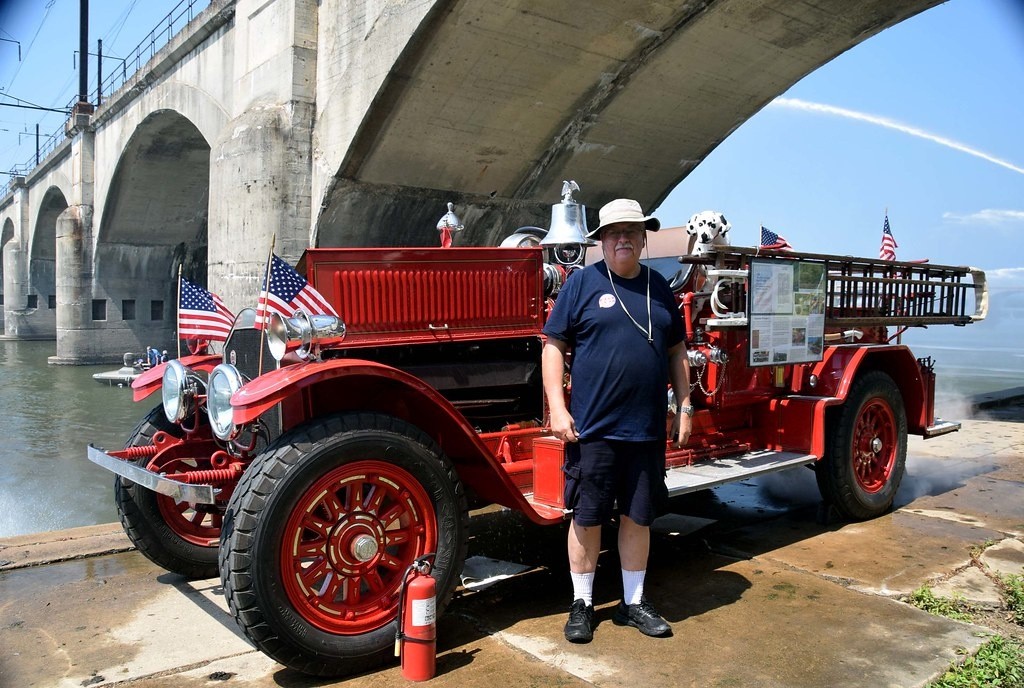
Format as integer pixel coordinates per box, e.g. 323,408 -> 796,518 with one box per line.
614,599 -> 671,636
565,599 -> 594,643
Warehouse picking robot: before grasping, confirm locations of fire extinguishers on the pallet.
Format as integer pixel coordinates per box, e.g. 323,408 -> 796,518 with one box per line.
394,552 -> 438,682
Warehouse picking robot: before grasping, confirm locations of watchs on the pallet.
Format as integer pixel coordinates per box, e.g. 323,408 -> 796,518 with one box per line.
676,405 -> 694,417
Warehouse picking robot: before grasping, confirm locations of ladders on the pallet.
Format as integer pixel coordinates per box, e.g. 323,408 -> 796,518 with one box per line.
675,243 -> 990,330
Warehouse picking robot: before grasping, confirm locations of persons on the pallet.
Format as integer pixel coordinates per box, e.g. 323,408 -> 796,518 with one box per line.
540,198 -> 694,642
146,344 -> 171,371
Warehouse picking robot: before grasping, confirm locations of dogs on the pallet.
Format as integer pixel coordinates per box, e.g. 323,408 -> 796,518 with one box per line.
685,210 -> 732,325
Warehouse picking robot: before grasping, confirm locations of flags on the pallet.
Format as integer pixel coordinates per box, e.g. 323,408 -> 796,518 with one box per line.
255,250 -> 341,337
760,225 -> 795,259
180,275 -> 241,344
880,216 -> 899,267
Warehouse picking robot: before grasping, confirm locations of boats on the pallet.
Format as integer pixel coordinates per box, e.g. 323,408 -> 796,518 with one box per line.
90,346 -> 161,388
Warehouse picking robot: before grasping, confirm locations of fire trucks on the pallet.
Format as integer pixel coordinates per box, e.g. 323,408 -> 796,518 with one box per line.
84,179 -> 991,676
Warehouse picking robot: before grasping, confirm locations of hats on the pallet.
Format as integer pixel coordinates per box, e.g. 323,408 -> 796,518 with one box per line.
163,350 -> 168,354
585,199 -> 661,241
147,346 -> 151,351
